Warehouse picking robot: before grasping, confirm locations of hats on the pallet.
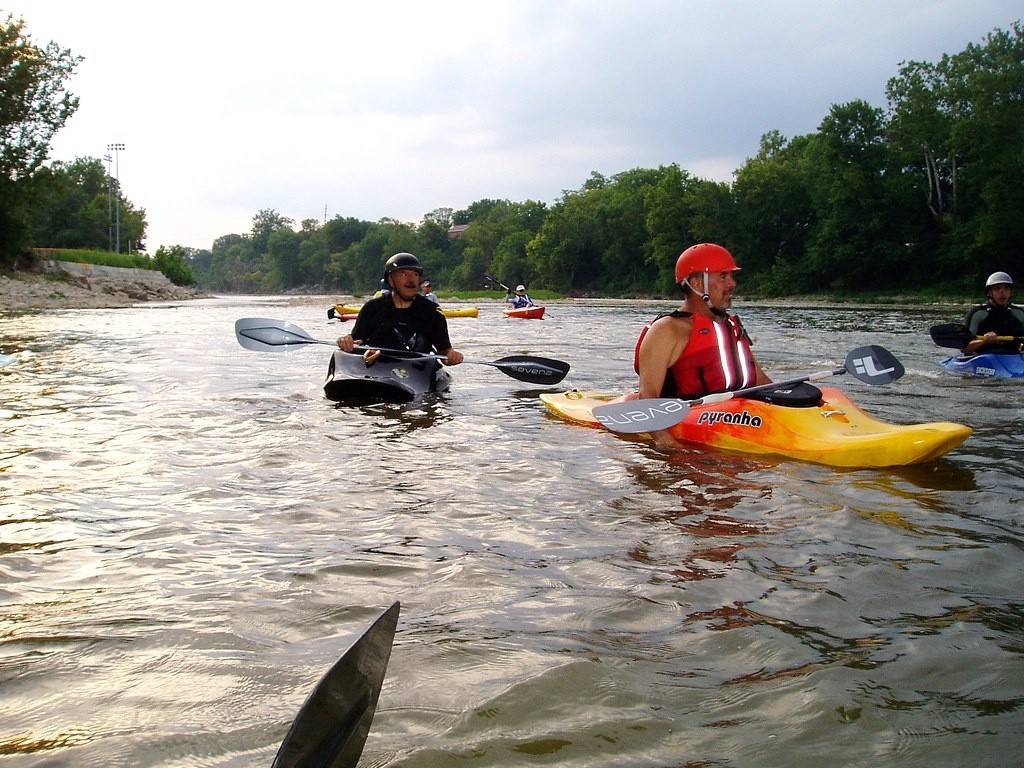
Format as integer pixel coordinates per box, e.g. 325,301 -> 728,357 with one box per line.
421,281 -> 431,287
516,285 -> 525,291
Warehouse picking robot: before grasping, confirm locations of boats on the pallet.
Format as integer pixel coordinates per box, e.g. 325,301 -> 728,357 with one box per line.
503,307 -> 545,319
938,352 -> 1024,378
540,386 -> 974,469
327,304 -> 478,323
323,350 -> 453,404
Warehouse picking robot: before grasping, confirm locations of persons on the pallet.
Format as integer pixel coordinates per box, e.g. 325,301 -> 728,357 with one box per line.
336,253 -> 463,366
633,243 -> 772,399
373,278 -> 389,298
963,272 -> 1024,356
421,281 -> 440,306
506,285 -> 532,308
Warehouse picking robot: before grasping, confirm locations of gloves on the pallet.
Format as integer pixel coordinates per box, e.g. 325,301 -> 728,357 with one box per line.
508,289 -> 511,294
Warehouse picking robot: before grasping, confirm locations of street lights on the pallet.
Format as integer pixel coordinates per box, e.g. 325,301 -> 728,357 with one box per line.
103,154 -> 112,251
107,144 -> 125,253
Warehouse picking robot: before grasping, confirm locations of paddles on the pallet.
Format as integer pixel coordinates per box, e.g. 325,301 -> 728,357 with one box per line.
928,322 -> 1023,349
271,598 -> 405,767
234,317 -> 572,385
482,271 -> 551,317
592,342 -> 907,435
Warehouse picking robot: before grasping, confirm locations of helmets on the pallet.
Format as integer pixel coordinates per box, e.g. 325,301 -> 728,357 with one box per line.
675,243 -> 742,283
379,279 -> 389,289
986,271 -> 1013,287
384,253 -> 423,285
